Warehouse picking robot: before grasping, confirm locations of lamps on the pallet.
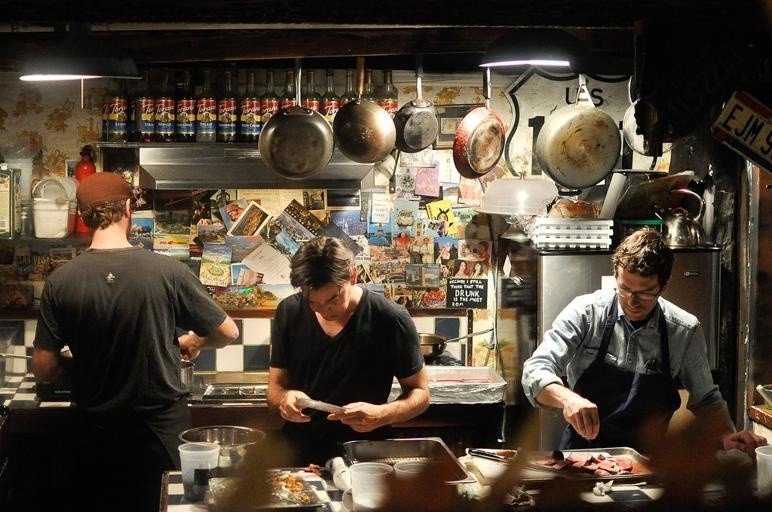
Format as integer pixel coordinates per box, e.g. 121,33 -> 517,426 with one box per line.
18,21 -> 144,84
478,24 -> 575,70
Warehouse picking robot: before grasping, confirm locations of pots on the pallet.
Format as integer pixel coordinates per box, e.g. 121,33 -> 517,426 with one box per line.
334,57 -> 401,163
177,360 -> 196,394
263,61 -> 334,181
415,325 -> 495,361
1,348 -> 74,363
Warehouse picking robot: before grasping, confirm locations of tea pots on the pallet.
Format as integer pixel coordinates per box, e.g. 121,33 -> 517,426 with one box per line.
651,189 -> 708,248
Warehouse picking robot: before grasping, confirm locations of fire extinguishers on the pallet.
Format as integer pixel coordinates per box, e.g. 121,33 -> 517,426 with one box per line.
74,144 -> 97,235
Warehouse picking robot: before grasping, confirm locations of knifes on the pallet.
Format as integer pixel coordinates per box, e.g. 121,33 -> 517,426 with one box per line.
466,445 -> 565,475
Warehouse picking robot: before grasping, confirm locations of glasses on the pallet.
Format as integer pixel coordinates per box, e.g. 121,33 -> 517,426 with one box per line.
614,284 -> 661,302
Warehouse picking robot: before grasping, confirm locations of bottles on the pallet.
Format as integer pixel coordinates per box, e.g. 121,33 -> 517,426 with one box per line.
264,61 -> 298,145
256,52 -> 681,197
325,456 -> 353,511
302,62 -> 400,130
19,202 -> 32,236
101,62 -> 262,145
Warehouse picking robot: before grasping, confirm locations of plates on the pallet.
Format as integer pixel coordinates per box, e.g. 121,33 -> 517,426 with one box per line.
210,471 -> 323,511
31,178 -> 68,200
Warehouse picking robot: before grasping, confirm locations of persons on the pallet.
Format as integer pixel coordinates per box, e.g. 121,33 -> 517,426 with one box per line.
521,225 -> 767,458
267,235 -> 430,468
32,172 -> 239,512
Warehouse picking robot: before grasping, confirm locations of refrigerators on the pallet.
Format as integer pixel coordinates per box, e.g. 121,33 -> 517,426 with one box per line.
492,234 -> 727,449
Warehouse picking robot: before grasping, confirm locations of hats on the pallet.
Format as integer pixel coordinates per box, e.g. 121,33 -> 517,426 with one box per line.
75,171 -> 137,210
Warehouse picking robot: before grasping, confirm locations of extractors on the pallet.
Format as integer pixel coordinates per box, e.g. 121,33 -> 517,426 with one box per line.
133,141 -> 378,194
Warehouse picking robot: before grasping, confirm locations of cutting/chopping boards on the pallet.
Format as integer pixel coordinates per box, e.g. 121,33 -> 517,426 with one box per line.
465,449 -> 621,480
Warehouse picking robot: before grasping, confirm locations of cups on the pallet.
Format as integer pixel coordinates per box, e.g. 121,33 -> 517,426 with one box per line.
391,461 -> 430,493
762,384 -> 772,410
349,459 -> 394,512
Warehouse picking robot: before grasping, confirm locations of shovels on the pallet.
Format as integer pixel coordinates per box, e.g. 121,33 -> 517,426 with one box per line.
465,447 -> 596,491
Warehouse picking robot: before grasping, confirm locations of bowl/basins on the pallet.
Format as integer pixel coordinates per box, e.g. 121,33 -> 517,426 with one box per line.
178,423 -> 266,479
469,176 -> 560,217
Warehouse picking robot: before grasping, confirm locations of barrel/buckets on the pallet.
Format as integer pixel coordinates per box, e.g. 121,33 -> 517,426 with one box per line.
393,461 -> 425,500
349,462 -> 393,508
33,199 -> 68,238
